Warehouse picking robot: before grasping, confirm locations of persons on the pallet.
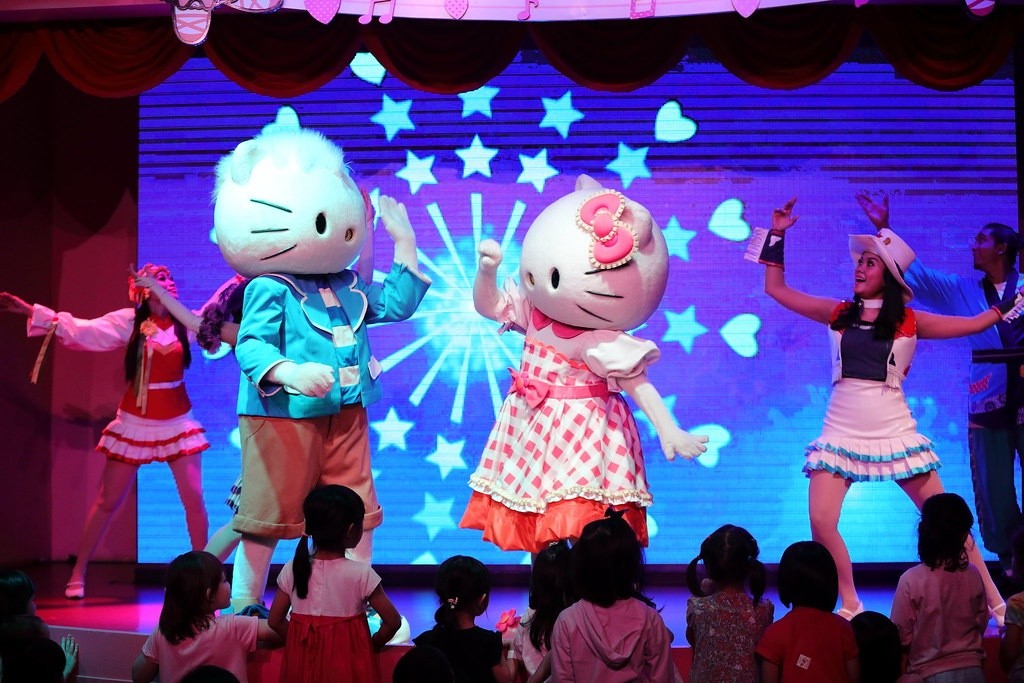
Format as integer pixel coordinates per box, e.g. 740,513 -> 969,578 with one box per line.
0,261 -> 247,599
754,196 -> 1023,630
0,485 -> 1024,683
853,195 -> 1023,597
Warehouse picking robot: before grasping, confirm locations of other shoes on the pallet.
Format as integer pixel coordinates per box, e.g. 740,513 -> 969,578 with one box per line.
64,582 -> 85,598
840,601 -> 863,622
987,602 -> 1007,627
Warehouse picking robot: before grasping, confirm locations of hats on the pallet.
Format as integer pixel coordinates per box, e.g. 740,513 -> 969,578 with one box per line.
848,228 -> 916,304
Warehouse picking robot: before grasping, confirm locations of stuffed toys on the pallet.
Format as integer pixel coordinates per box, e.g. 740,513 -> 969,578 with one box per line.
458,175 -> 710,645
213,121 -> 429,648
